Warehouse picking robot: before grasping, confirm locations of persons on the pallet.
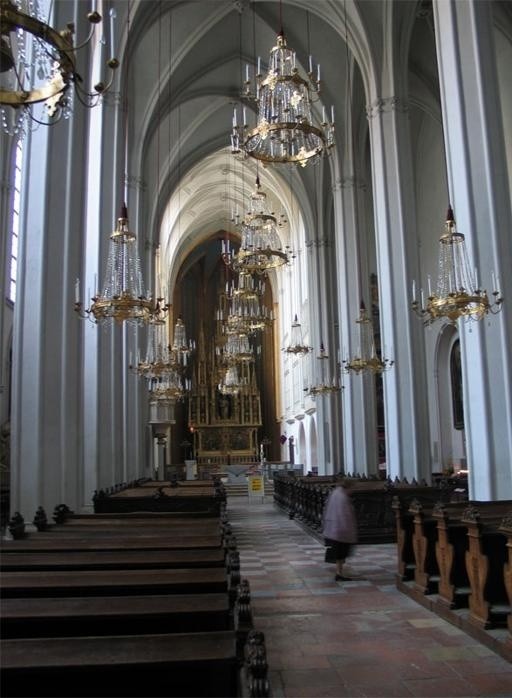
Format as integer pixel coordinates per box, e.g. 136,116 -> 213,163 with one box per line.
321,477 -> 360,582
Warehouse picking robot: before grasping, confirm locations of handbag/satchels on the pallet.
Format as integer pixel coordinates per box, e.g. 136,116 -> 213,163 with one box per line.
324,547 -> 345,562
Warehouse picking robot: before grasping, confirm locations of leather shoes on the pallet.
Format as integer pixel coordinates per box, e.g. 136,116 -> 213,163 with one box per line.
335,575 -> 352,581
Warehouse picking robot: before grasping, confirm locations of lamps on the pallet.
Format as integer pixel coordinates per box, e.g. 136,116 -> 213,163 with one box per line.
1,0 -> 505,403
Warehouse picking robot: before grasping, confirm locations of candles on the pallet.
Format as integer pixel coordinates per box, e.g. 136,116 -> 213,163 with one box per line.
261,444 -> 264,454
